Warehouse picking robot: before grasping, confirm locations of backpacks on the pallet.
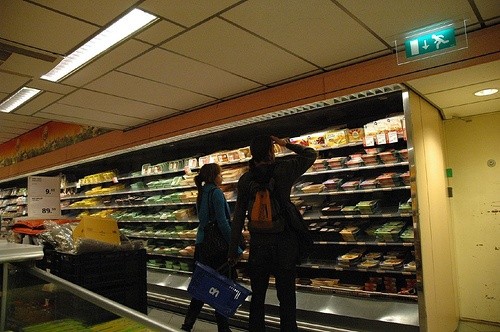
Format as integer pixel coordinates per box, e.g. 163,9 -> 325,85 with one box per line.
240,159 -> 287,234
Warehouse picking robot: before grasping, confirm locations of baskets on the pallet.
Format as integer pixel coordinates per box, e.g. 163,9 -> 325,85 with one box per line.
187,260 -> 252,319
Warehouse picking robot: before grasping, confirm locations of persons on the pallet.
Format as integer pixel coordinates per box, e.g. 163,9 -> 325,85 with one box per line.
178,163 -> 243,332
232,136 -> 317,332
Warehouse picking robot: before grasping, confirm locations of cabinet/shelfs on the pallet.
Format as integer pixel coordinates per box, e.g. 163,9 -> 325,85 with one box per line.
0,135 -> 418,298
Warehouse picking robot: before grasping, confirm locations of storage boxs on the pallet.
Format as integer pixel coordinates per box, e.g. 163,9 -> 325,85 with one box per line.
72,216 -> 120,252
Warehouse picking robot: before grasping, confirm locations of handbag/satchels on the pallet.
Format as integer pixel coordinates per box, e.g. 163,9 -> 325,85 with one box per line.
203,221 -> 236,256
